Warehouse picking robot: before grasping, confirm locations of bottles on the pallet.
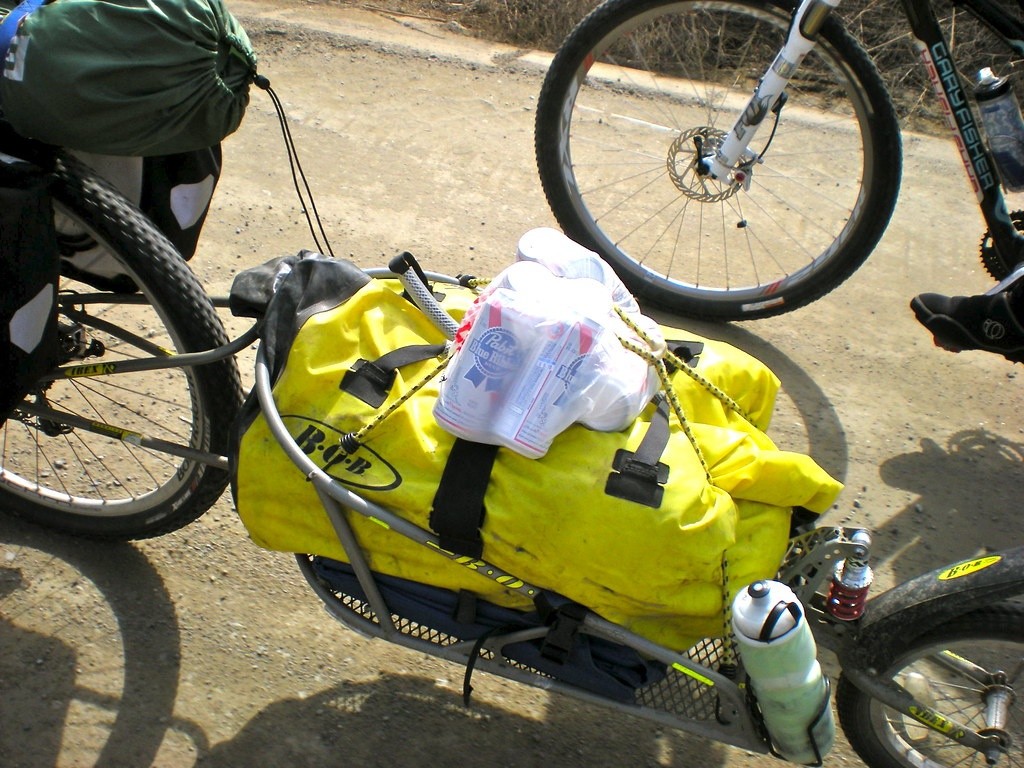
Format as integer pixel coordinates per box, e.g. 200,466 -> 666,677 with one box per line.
730,579 -> 834,763
973,67 -> 1024,193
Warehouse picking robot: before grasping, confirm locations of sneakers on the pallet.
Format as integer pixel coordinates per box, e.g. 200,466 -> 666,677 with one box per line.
909,284 -> 1024,362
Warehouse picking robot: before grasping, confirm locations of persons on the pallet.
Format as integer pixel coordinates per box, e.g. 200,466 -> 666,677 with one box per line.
911,280 -> 1024,362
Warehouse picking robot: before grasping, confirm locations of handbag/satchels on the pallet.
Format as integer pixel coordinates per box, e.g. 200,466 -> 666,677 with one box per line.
0,0 -> 256,157
227,253 -> 846,661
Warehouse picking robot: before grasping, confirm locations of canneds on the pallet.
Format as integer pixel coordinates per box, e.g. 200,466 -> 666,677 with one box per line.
433,227 -> 658,458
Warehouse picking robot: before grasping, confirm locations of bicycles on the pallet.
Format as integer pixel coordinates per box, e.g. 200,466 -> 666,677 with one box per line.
1,120 -> 245,545
535,0 -> 1024,364
